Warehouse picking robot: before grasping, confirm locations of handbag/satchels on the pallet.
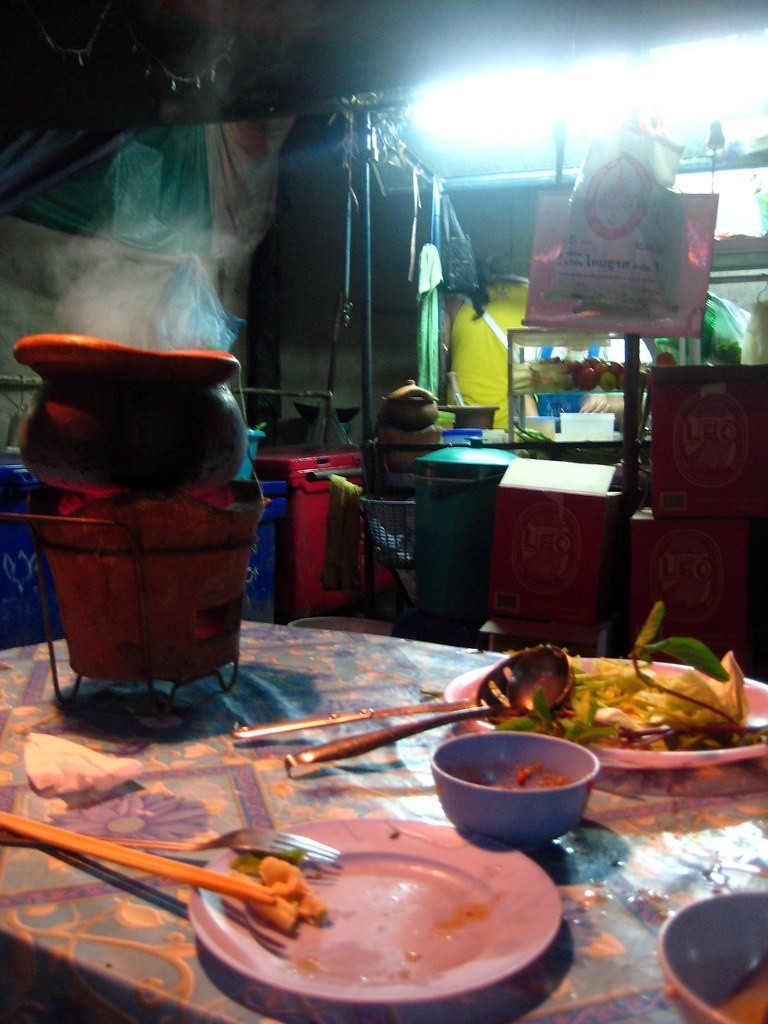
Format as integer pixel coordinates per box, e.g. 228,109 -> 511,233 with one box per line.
523,130 -> 719,337
436,193 -> 479,292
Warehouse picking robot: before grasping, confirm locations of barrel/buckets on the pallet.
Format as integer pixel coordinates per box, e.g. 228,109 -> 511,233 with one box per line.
27,464 -> 264,688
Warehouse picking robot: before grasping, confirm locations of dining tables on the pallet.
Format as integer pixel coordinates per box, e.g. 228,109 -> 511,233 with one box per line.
0,618 -> 767,1024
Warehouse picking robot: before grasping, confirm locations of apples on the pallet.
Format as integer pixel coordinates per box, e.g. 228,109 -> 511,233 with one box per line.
565,350 -> 674,390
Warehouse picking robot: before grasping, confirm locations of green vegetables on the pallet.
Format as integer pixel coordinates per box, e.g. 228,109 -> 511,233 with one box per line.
492,600 -> 768,754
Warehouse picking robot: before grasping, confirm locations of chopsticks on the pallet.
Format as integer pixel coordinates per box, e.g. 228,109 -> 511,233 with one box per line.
0,811 -> 278,905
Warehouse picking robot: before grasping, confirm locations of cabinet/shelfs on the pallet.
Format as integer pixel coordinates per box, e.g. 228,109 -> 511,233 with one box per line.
504,323 -> 700,455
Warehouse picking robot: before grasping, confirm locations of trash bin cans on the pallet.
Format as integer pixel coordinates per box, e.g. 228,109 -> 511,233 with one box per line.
254,447 -> 368,622
1,461 -> 70,652
237,479 -> 288,626
407,446 -> 522,645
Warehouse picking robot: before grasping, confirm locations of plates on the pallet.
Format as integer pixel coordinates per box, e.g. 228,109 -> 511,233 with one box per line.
445,659 -> 768,767
188,820 -> 562,1003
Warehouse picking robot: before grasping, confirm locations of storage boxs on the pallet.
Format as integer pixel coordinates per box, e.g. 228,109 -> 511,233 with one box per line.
627,363 -> 768,674
478,458 -> 623,661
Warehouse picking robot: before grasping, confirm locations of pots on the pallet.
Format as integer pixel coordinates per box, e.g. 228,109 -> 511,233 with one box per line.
13,334 -> 243,494
377,380 -> 439,431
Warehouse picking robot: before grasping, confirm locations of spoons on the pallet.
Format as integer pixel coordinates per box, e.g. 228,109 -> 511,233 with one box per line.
284,646 -> 575,777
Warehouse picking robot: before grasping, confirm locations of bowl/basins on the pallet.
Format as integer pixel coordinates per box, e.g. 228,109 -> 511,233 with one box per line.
430,731 -> 599,853
657,892 -> 767,1024
288,616 -> 394,641
442,428 -> 483,446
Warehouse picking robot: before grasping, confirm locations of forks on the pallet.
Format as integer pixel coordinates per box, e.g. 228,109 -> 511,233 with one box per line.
0,828 -> 342,866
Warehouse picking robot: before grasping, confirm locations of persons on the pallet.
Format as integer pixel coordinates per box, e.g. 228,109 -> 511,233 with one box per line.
439,282 -> 529,430
519,334 -> 654,428
646,290 -> 753,391
